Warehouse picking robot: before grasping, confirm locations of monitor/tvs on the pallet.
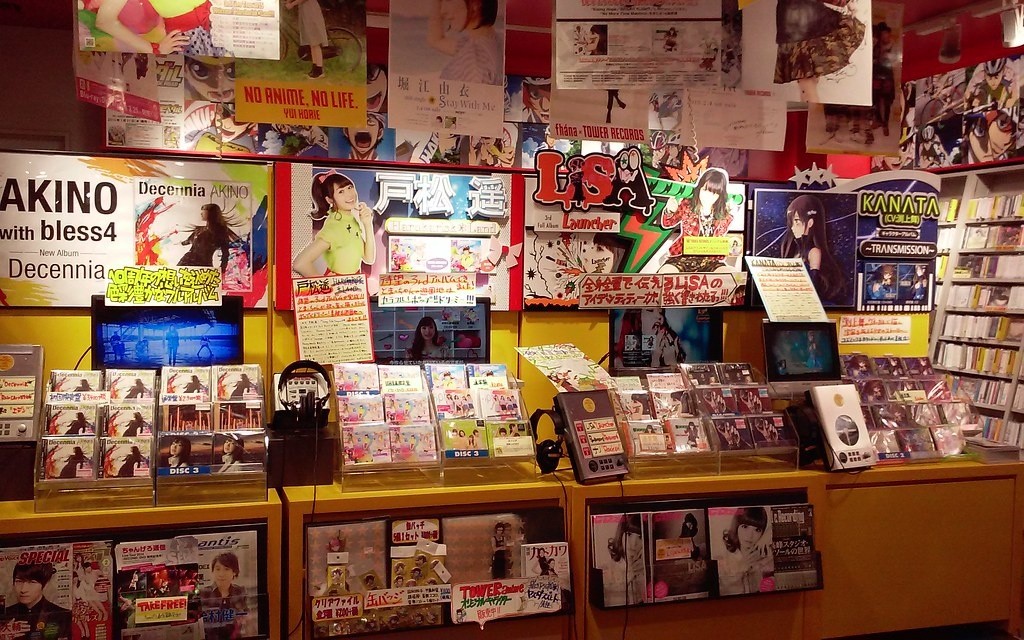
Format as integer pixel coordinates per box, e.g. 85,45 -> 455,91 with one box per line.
369,296 -> 491,364
762,318 -> 843,394
607,307 -> 723,379
91,295 -> 244,376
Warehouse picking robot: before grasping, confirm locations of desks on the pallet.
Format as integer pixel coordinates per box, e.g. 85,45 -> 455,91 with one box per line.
812,456 -> 1023,637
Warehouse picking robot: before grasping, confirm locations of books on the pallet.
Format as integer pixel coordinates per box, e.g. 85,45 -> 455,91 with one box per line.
930,193 -> 1024,461
45,363 -> 268,480
841,352 -> 983,461
592,503 -> 817,610
610,363 -> 789,454
332,360 -> 534,465
0,529 -> 257,640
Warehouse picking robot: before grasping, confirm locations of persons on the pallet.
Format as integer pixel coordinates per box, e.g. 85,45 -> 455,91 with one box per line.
582,232 -> 633,273
658,168 -> 737,272
292,170 -> 376,276
217,434 -> 244,472
200,550 -> 249,639
663,27 -> 678,51
0,556 -> 71,640
584,24 -> 607,56
429,0 -> 503,86
286,0 -> 328,79
774,0 -> 866,102
597,514 -> 645,606
110,323 -> 214,366
537,548 -> 559,576
405,316 -> 453,358
73,553 -> 109,639
714,507 -> 773,595
176,204 -> 230,283
169,437 -> 191,467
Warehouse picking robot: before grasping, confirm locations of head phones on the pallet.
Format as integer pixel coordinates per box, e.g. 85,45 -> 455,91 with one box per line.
527,409 -> 565,474
279,360 -> 331,427
782,405 -> 820,467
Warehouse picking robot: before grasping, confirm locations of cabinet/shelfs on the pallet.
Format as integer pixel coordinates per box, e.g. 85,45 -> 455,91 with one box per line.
930,163 -> 1023,464
282,464 -> 573,640
552,454 -> 805,639
1,480 -> 281,639
